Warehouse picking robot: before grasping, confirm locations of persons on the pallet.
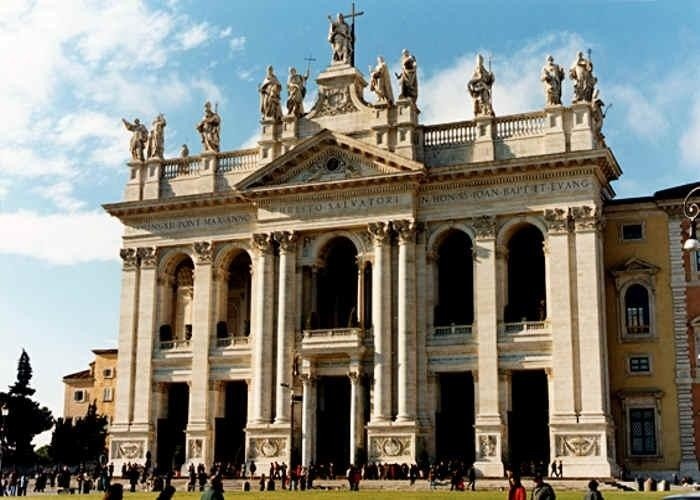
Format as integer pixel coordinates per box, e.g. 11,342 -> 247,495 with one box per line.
347,460 -> 476,492
324,11 -> 353,64
287,66 -> 311,119
256,63 -> 284,126
368,54 -> 396,106
569,52 -> 600,105
539,55 -> 566,106
1,449 -> 182,500
505,454 -> 603,499
146,113 -> 167,159
188,462 -> 334,499
121,116 -> 149,164
468,51 -> 497,117
196,100 -> 221,154
395,48 -> 418,102
